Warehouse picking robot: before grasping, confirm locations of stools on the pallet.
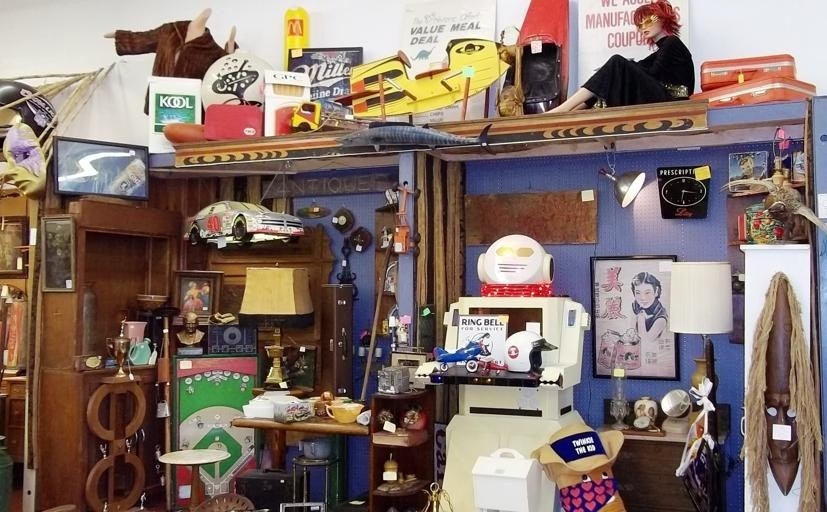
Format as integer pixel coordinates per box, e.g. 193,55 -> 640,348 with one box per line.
292,456 -> 335,512
158,448 -> 231,512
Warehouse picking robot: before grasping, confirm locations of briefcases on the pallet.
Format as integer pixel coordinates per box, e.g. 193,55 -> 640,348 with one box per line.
689,75 -> 816,108
701,54 -> 796,91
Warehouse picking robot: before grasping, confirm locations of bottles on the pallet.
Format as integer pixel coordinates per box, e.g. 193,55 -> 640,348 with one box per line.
128,338 -> 152,366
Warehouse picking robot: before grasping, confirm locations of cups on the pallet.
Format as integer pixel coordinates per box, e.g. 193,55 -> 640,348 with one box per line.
124,322 -> 148,348
384,460 -> 399,484
327,403 -> 364,423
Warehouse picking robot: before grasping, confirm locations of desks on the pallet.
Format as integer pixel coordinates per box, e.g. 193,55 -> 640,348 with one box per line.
232,417 -> 369,469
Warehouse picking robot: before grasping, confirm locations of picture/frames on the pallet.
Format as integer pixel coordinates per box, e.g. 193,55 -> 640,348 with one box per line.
53,136 -> 151,200
172,269 -> 225,327
287,48 -> 363,102
0,215 -> 28,279
590,255 -> 681,382
40,216 -> 76,293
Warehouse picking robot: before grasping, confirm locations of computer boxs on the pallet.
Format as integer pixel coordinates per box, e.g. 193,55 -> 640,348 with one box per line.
235,469 -> 311,512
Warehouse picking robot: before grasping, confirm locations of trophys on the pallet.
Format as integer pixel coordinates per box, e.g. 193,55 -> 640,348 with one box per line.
105,337 -> 137,377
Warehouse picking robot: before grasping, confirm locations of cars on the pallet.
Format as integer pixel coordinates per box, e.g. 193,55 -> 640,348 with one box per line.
183,201 -> 305,249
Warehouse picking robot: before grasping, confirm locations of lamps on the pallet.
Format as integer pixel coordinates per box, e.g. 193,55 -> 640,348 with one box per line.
670,262 -> 732,408
238,266 -> 315,391
599,168 -> 646,209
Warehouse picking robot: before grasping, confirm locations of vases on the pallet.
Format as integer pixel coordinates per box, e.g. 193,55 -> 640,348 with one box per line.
634,396 -> 658,430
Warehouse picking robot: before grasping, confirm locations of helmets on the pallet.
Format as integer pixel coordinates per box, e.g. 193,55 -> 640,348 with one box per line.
201,52 -> 274,113
505,331 -> 558,373
0,80 -> 58,154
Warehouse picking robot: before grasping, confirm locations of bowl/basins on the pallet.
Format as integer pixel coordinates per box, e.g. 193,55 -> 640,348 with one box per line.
135,293 -> 169,311
298,438 -> 333,459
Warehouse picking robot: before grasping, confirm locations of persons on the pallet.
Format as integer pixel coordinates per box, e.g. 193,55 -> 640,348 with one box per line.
542,0 -> 696,114
104,5 -> 239,116
630,271 -> 668,345
176,311 -> 205,345
183,281 -> 204,312
731,157 -> 765,196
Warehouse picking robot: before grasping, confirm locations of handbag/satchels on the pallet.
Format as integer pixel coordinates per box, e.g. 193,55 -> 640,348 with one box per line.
680,432 -> 721,512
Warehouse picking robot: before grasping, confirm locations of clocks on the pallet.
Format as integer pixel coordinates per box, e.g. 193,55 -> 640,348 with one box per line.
656,165 -> 712,220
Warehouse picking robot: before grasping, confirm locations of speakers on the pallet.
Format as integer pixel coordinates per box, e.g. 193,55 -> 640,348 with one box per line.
320,284 -> 353,399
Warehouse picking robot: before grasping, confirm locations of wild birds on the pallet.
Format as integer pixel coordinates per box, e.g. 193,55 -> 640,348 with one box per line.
720,180 -> 827,234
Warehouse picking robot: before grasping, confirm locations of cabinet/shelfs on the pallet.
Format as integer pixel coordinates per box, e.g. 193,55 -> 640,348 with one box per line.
369,383 -> 437,512
2,380 -> 25,467
37,202 -> 173,512
727,182 -> 806,344
373,203 -> 397,333
596,423 -> 728,512
